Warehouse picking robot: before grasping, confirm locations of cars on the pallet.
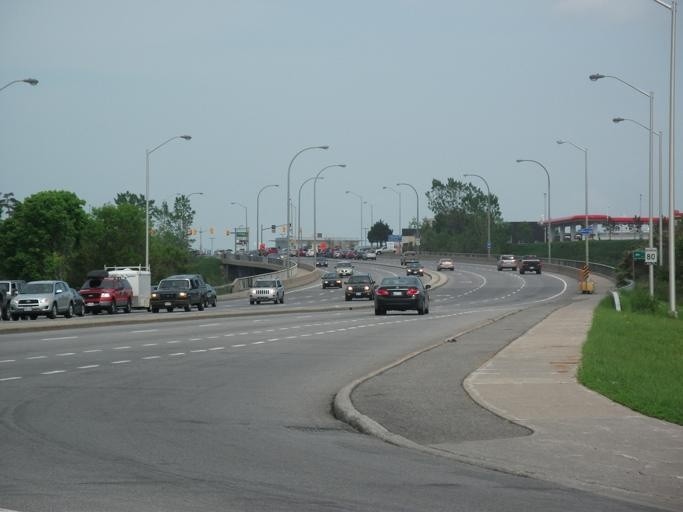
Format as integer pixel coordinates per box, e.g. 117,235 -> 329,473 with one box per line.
69,287 -> 86,316
399,250 -> 424,276
203,283 -> 217,307
320,272 -> 342,289
334,261 -> 354,278
370,275 -> 431,316
436,257 -> 455,271
216,246 -> 396,267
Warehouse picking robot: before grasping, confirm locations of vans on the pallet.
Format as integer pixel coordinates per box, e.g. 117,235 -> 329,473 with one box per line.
0,279 -> 26,320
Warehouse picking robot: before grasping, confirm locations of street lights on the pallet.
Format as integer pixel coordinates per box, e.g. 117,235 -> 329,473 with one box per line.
555,138 -> 590,284
343,180 -> 420,249
588,72 -> 655,303
610,115 -> 663,270
184,173 -> 296,258
296,176 -> 324,257
312,164 -> 347,270
0,79 -> 39,90
284,145 -> 329,279
462,173 -> 491,258
144,133 -> 192,270
515,158 -> 552,262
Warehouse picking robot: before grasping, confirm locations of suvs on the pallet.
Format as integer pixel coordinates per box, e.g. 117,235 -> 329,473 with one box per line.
149,273 -> 208,314
248,277 -> 284,305
343,273 -> 377,302
7,280 -> 74,321
495,254 -> 517,272
517,254 -> 542,274
77,277 -> 133,316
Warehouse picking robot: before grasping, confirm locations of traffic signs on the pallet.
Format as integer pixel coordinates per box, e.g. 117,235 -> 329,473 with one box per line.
643,247 -> 658,264
